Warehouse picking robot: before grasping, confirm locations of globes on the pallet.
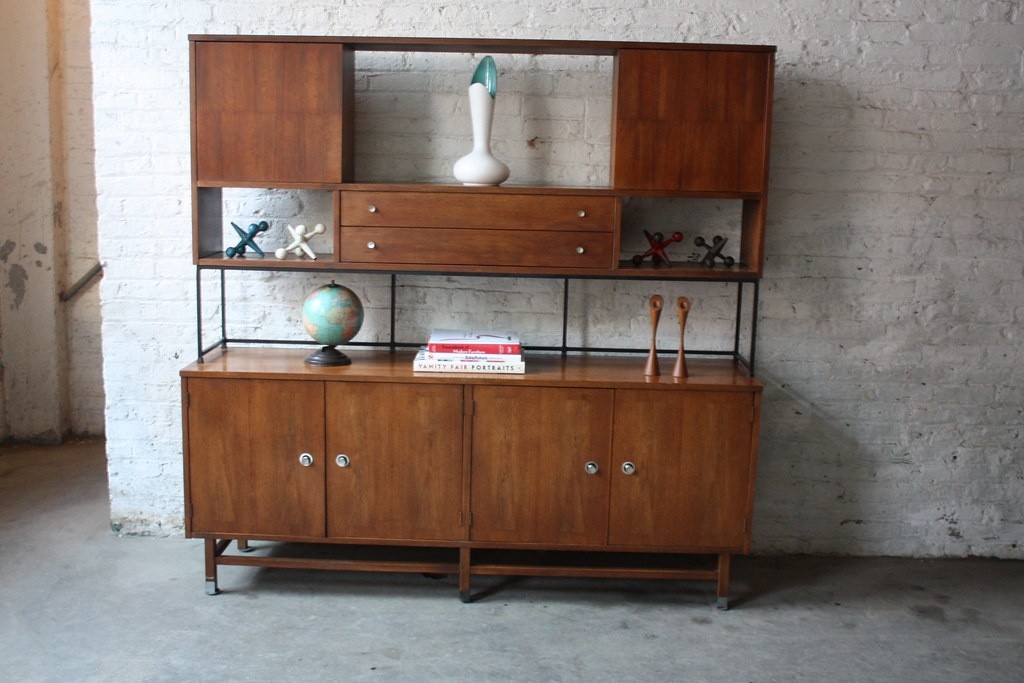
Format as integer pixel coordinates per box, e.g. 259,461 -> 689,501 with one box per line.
301,279 -> 364,368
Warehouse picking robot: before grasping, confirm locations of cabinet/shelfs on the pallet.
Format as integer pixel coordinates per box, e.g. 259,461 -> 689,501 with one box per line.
177,33 -> 778,610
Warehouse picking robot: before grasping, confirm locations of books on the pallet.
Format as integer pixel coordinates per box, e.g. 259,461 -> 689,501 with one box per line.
413,333 -> 525,373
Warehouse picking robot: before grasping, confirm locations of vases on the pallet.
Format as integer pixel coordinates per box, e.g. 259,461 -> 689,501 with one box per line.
452,54 -> 513,186
